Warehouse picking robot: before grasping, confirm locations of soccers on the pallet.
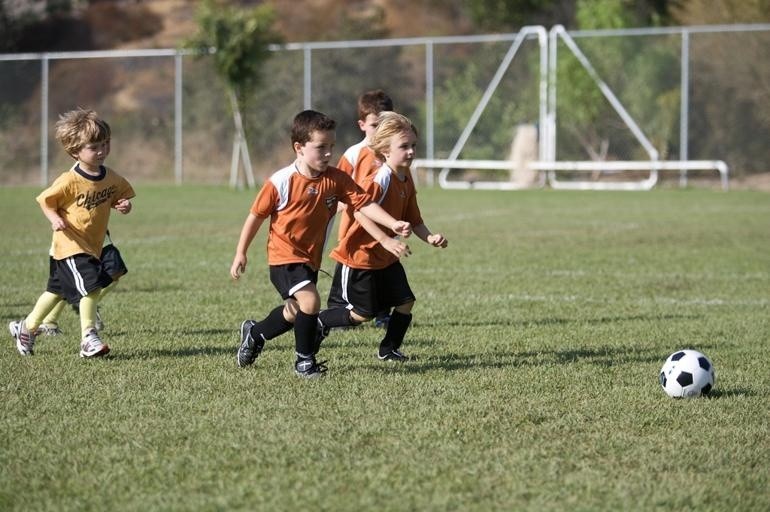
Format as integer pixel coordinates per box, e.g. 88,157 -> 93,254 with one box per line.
659,349 -> 715,399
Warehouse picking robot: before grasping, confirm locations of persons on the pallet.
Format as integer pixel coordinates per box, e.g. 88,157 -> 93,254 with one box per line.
327,90 -> 393,330
313,110 -> 448,362
9,107 -> 136,359
33,120 -> 128,336
230,109 -> 412,378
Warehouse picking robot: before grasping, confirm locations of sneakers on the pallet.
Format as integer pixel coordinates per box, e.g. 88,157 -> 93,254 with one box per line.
317,310 -> 330,354
95,311 -> 105,330
34,323 -> 63,337
80,327 -> 110,358
237,319 -> 265,371
375,350 -> 407,363
294,355 -> 322,381
375,316 -> 391,330
9,319 -> 34,357
